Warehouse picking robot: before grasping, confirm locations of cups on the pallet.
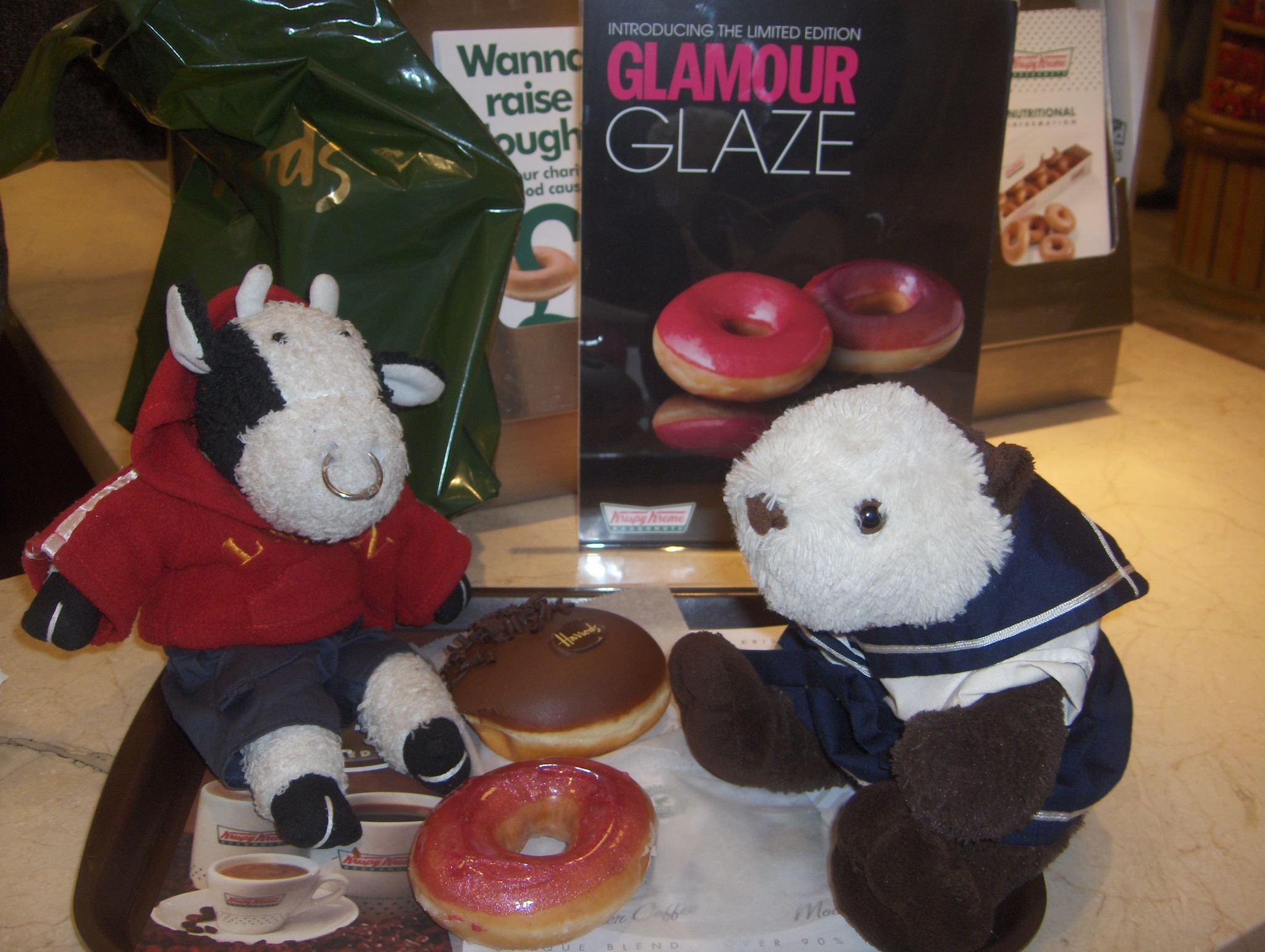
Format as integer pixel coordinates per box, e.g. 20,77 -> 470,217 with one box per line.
208,850 -> 350,935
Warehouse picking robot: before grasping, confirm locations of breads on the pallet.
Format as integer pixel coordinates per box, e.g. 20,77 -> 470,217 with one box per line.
428,595 -> 680,769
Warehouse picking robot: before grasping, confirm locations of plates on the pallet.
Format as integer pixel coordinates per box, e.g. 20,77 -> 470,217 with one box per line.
151,888 -> 360,946
72,588 -> 876,952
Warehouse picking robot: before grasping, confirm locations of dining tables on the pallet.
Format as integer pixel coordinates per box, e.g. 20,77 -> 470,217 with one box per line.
3,319 -> 1265,952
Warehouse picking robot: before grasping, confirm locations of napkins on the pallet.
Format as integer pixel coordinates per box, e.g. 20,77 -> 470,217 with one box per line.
419,623 -> 879,951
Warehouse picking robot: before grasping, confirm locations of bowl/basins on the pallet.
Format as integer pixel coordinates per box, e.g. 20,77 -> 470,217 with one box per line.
188,780 -> 309,888
308,791 -> 449,924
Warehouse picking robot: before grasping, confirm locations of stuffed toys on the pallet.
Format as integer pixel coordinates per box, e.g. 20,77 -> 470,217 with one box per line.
19,263 -> 479,846
667,382 -> 1151,952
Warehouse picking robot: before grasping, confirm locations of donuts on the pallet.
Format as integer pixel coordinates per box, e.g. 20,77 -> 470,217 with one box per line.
639,255 -> 967,461
394,753 -> 668,950
